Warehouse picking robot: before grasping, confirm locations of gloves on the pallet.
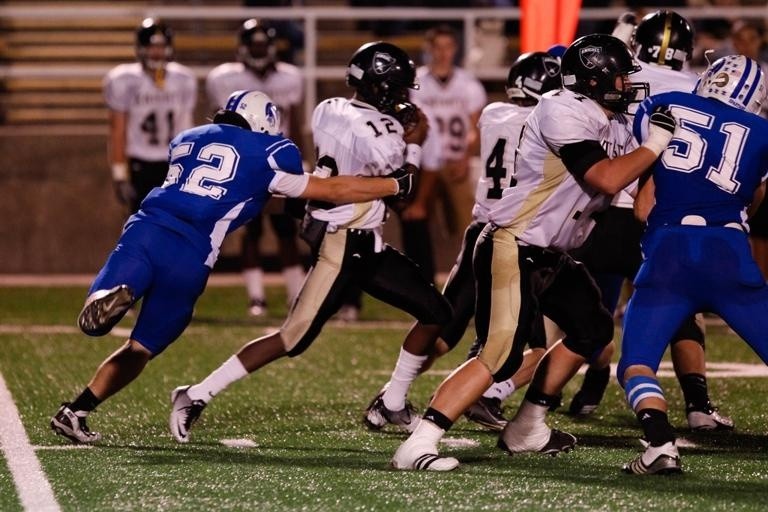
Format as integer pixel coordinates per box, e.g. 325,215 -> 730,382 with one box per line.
113,181 -> 137,205
283,198 -> 307,218
384,168 -> 414,199
640,105 -> 675,157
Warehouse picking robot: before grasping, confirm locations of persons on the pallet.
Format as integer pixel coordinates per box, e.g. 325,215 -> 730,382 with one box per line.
49,88 -> 421,443
102,16 -> 199,320
390,8 -> 766,477
168,40 -> 455,446
207,16 -> 306,320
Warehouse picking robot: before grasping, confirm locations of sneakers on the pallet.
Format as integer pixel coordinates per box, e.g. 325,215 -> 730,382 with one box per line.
686,410 -> 733,433
364,391 -> 422,431
332,304 -> 360,319
50,403 -> 101,444
246,298 -> 268,318
621,437 -> 680,476
77,284 -> 135,335
390,436 -> 459,472
462,396 -> 506,432
570,365 -> 609,414
168,385 -> 206,445
496,421 -> 575,455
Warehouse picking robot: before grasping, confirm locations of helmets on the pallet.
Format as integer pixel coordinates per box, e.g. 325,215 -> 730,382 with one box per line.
235,17 -> 277,69
690,54 -> 766,115
223,89 -> 281,137
627,10 -> 693,71
344,41 -> 419,106
505,52 -> 561,106
560,32 -> 649,116
135,18 -> 173,69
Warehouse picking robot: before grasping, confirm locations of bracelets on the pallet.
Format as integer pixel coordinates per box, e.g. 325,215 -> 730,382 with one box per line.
110,160 -> 131,184
